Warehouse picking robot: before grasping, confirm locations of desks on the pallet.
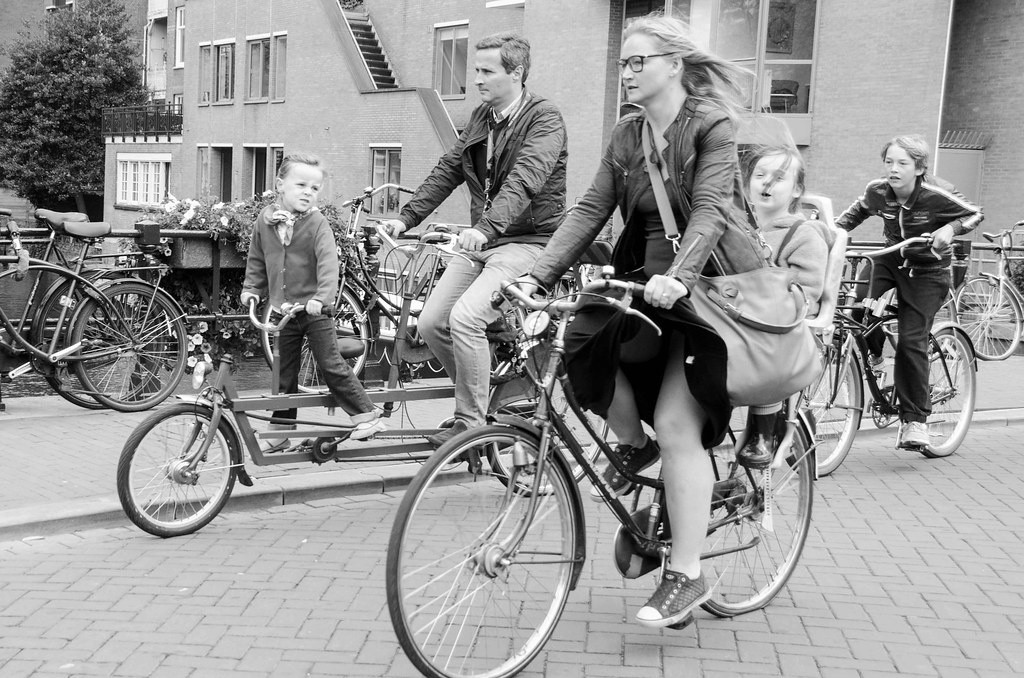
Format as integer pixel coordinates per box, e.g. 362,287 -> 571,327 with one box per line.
771,93 -> 796,112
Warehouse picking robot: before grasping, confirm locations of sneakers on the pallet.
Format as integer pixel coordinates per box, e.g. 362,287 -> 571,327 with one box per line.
862,355 -> 886,382
902,421 -> 929,446
701,403 -> 734,448
634,569 -> 712,631
737,414 -> 786,468
588,434 -> 660,504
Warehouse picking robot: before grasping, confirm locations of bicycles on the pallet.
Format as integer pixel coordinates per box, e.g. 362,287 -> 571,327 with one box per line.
260,184 -> 529,394
0,208 -> 189,412
785,232 -> 978,477
955,221 -> 1024,362
388,265 -> 820,678
116,218 -> 610,538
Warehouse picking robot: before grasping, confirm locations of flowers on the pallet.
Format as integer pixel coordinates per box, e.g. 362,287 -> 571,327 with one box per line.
120,187 -> 280,271
154,272 -> 268,375
321,202 -> 370,300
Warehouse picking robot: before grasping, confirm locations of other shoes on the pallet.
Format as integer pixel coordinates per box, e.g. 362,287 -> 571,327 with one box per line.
350,408 -> 386,439
428,420 -> 484,450
261,438 -> 292,454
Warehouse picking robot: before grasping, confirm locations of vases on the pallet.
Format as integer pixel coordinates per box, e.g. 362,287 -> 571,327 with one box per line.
172,232 -> 247,270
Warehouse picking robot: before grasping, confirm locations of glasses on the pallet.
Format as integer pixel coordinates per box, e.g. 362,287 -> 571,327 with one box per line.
616,52 -> 677,73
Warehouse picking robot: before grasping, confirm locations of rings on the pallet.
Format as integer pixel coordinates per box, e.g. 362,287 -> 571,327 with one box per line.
662,291 -> 671,300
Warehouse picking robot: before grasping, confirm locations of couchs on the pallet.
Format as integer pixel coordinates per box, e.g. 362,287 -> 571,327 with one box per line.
770,78 -> 799,112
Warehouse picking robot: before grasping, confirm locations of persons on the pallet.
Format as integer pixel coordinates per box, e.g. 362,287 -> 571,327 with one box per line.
240,154 -> 385,453
735,119 -> 837,469
835,134 -> 986,448
510,11 -> 757,627
383,32 -> 569,446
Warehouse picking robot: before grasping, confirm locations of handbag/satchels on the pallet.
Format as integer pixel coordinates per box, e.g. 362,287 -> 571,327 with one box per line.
686,264 -> 823,406
